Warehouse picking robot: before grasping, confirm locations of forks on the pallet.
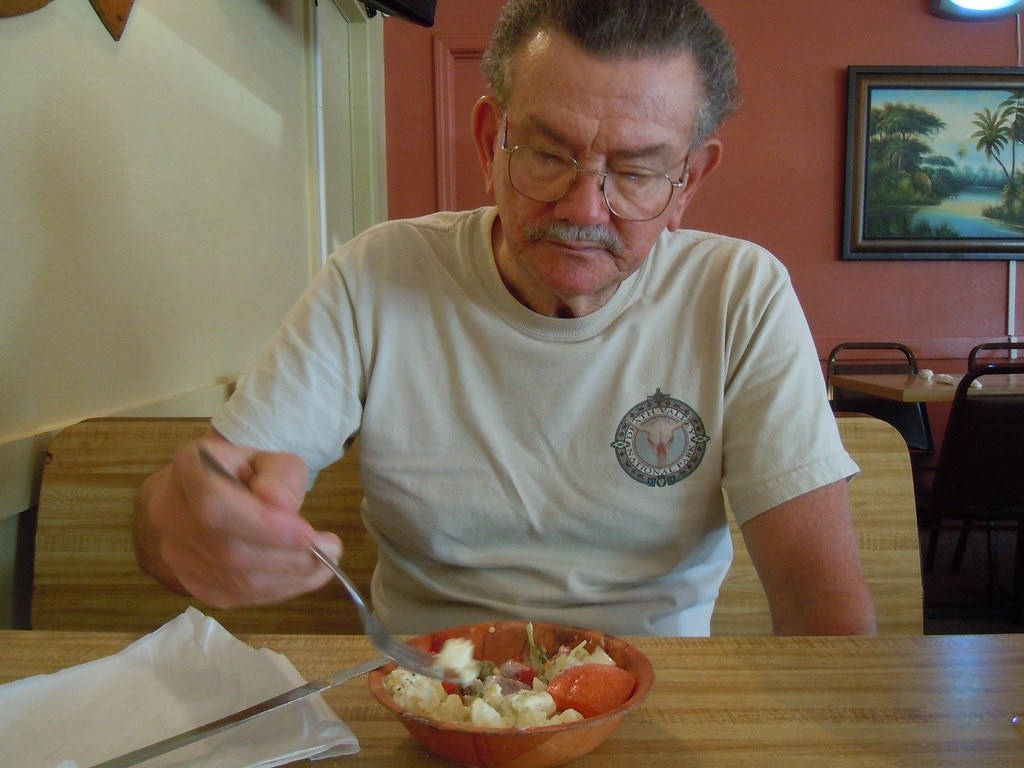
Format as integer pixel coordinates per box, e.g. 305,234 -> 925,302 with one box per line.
197,444 -> 449,680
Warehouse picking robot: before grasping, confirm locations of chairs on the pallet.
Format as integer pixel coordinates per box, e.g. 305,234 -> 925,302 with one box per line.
30,407 -> 925,639
825,342 -> 935,532
967,341 -> 1023,592
922,362 -> 1024,598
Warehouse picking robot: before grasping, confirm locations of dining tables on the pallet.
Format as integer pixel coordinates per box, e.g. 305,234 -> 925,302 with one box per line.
2,632 -> 1024,768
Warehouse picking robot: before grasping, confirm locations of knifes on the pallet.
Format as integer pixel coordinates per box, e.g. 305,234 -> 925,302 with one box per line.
93,653 -> 395,768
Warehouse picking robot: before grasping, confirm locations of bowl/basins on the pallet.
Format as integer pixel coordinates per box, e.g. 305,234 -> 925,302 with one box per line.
369,619 -> 657,768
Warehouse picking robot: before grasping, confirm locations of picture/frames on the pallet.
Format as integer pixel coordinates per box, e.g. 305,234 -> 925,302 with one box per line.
841,66 -> 1024,261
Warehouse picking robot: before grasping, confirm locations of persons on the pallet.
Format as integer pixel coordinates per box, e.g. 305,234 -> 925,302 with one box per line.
133,0 -> 878,639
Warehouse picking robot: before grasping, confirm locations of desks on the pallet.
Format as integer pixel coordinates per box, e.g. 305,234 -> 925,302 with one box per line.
832,374 -> 1024,403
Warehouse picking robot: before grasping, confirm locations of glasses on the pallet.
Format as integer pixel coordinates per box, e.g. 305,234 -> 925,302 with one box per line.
502,105 -> 690,222
